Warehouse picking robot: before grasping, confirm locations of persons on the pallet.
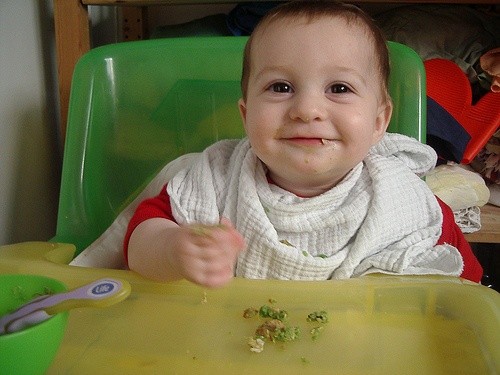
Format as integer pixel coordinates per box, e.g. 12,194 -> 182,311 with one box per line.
122,0 -> 484,288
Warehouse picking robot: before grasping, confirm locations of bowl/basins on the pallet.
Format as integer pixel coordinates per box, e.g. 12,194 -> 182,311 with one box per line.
0,273 -> 69,375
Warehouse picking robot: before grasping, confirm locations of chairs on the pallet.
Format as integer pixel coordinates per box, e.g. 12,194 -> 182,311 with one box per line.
47,35 -> 427,256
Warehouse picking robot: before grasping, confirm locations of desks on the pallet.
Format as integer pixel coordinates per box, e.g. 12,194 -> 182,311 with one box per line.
0,241 -> 500,375
